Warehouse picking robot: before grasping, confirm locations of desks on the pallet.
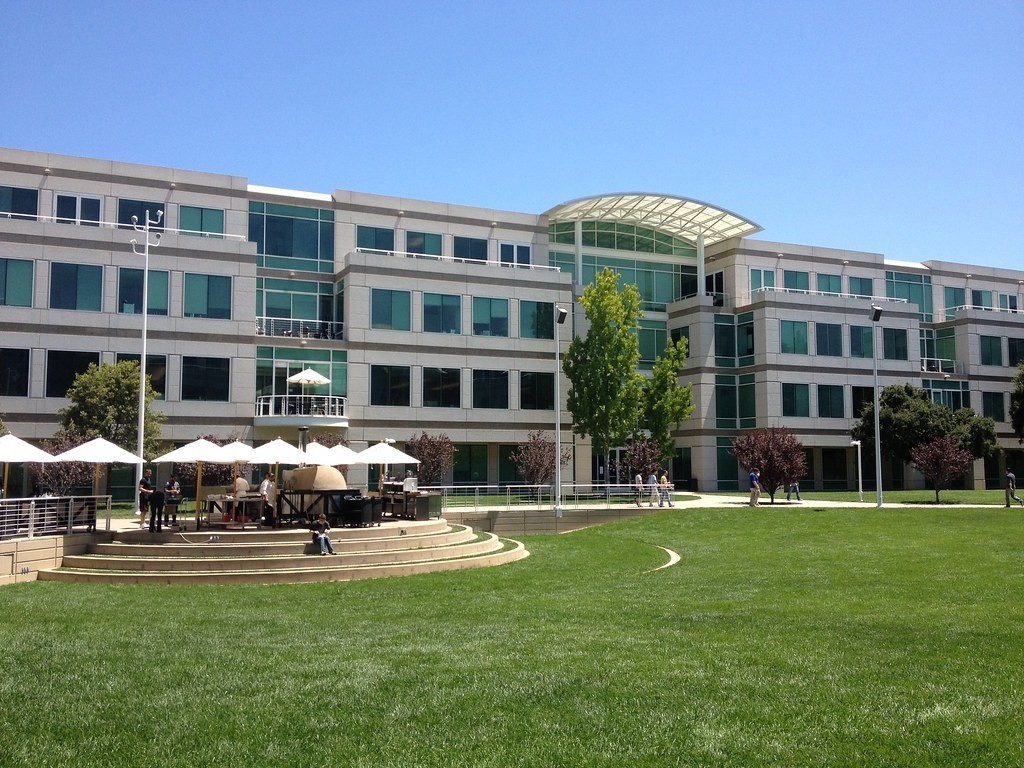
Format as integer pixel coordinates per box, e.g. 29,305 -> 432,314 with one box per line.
203,498 -> 262,529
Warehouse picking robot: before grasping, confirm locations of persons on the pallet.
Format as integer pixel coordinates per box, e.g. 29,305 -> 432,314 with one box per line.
164,474 -> 181,526
649,469 -> 661,507
139,469 -> 154,529
749,468 -> 761,508
1005,467 -> 1023,508
786,480 -> 803,501
259,472 -> 270,499
635,471 -> 644,507
658,470 -> 675,507
233,472 -> 250,515
310,514 -> 339,555
264,472 -> 278,525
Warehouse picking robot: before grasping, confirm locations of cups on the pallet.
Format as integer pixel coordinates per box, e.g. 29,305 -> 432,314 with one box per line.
338,537 -> 341,542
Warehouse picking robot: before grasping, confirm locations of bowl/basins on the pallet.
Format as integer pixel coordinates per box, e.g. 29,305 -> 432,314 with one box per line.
223,515 -> 231,522
237,516 -> 249,522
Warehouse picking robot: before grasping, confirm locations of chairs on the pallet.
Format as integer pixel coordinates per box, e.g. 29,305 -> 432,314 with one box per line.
281,327 -> 330,339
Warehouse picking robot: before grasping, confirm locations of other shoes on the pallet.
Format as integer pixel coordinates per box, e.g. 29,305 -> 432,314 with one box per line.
172,523 -> 180,526
669,505 -> 674,507
750,504 -> 759,507
1004,505 -> 1010,508
164,521 -> 168,526
330,552 -> 337,555
141,523 -> 150,528
1020,500 -> 1023,507
321,552 -> 326,556
660,506 -> 665,507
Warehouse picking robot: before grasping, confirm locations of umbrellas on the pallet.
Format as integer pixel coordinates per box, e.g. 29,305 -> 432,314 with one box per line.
55,436 -> 147,529
223,438 -> 254,523
254,435 -> 311,522
359,440 -> 421,498
151,437 -> 250,524
299,439 -> 362,467
287,367 -> 331,415
0,431 -> 62,506
